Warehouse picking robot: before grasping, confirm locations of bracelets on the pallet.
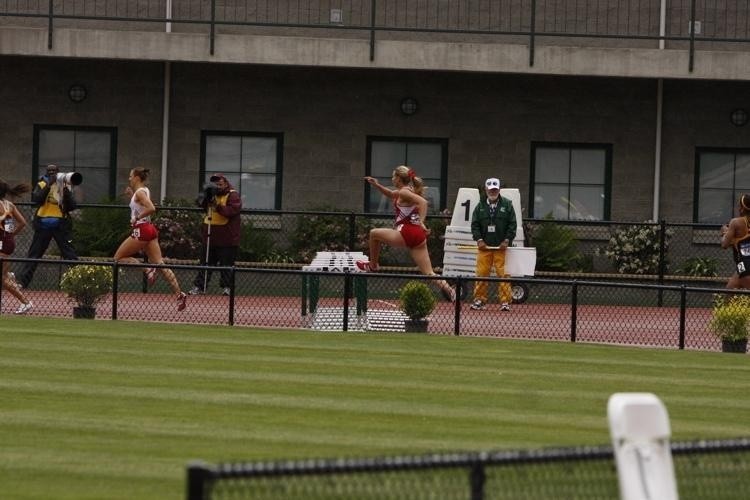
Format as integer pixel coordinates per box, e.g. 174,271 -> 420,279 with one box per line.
477,239 -> 483,242
378,184 -> 384,189
135,215 -> 140,219
721,232 -> 727,236
505,239 -> 509,242
417,219 -> 425,222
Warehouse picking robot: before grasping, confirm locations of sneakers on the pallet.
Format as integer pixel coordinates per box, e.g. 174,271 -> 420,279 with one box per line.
176,292 -> 187,312
469,298 -> 487,310
13,301 -> 34,314
355,260 -> 380,273
145,268 -> 159,286
498,301 -> 510,312
448,289 -> 456,305
16,284 -> 24,291
7,272 -> 16,284
188,287 -> 204,295
222,287 -> 230,296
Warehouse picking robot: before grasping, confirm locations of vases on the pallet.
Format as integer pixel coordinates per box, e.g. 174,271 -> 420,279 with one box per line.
73,307 -> 96,319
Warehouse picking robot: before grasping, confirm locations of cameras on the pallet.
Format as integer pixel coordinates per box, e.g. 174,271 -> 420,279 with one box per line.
197,181 -> 222,207
56,171 -> 86,188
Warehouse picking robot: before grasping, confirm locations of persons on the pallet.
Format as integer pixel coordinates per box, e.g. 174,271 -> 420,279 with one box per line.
721,193 -> 750,290
188,173 -> 242,296
114,166 -> 187,310
356,166 -> 456,307
471,178 -> 516,312
15,164 -> 81,289
0,179 -> 33,314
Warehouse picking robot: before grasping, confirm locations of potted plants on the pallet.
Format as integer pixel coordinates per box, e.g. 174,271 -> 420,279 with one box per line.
707,294 -> 749,352
401,281 -> 433,332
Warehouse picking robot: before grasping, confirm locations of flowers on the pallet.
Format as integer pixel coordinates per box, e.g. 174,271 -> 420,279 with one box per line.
61,265 -> 124,307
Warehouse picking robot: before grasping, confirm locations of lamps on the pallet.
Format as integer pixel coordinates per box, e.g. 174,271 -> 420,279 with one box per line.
731,108 -> 748,127
69,84 -> 86,102
400,97 -> 417,114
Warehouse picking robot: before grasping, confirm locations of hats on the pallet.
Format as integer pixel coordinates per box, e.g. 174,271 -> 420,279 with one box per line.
485,178 -> 501,190
210,172 -> 226,182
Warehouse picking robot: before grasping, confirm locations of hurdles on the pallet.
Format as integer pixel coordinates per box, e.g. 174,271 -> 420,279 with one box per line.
300,251 -> 370,329
